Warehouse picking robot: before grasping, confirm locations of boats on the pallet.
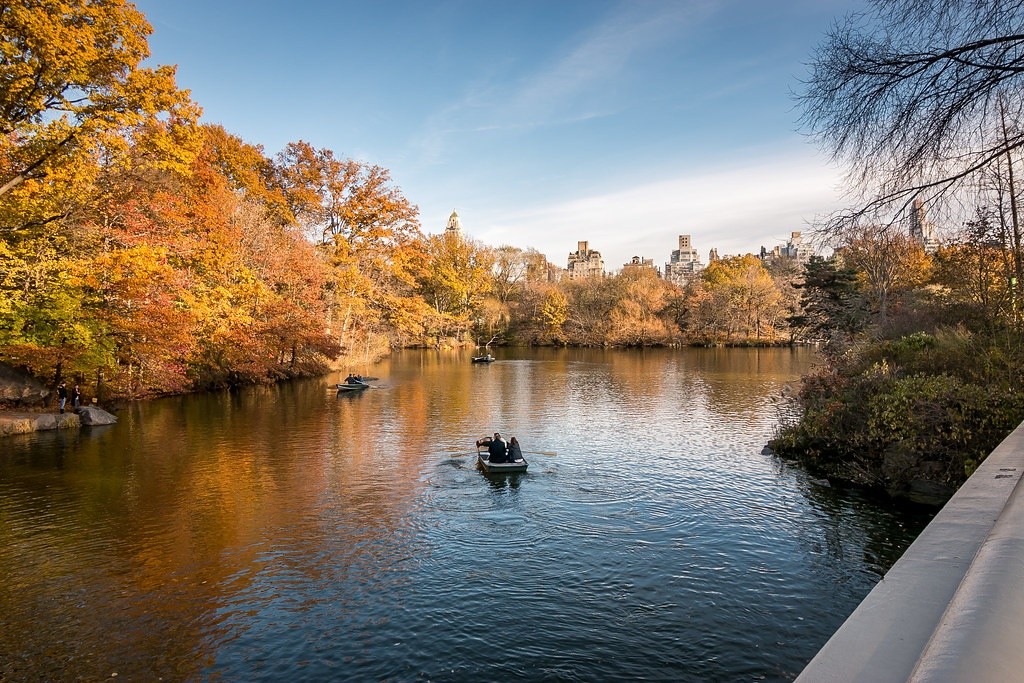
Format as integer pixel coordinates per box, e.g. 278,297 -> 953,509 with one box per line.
336,382 -> 370,391
471,356 -> 496,363
476,436 -> 530,474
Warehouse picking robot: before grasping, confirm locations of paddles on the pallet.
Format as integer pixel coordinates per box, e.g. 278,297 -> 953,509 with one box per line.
476,440 -> 559,457
355,380 -> 378,389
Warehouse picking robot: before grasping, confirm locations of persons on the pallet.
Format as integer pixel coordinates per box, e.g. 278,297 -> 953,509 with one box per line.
489,433 -> 523,463
344,374 -> 363,384
57,382 -> 81,414
486,353 -> 491,361
